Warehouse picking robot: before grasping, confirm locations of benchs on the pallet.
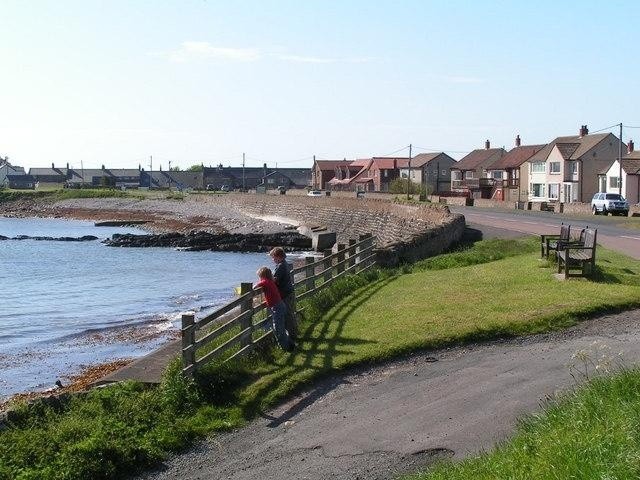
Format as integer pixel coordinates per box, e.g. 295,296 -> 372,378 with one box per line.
540,222 -> 598,279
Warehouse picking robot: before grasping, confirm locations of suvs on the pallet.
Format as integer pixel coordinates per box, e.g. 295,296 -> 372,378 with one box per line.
206,184 -> 216,191
591,193 -> 630,217
221,185 -> 230,192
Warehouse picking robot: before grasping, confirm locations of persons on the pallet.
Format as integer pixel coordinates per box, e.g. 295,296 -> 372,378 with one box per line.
269,247 -> 295,316
253,267 -> 295,353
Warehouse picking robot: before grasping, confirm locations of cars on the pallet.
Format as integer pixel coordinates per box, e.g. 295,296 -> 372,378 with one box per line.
307,191 -> 324,197
